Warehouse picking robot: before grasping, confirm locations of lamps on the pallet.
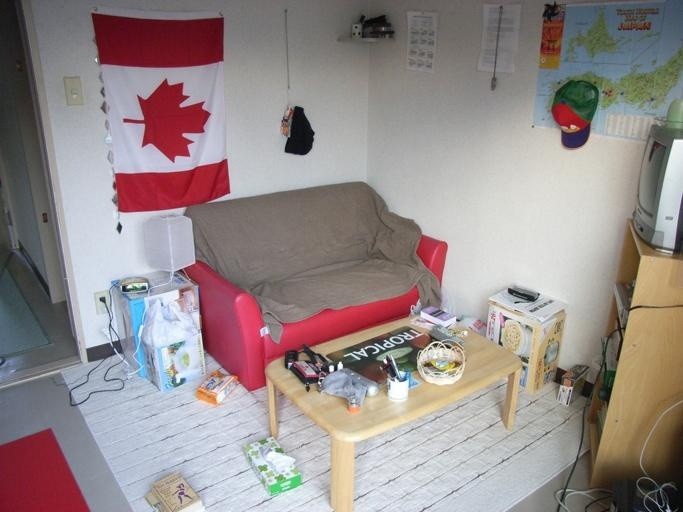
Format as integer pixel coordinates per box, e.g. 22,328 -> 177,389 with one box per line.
143,214 -> 195,285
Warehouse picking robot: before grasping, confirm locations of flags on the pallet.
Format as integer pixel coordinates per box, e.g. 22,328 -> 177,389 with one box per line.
90,5 -> 230,212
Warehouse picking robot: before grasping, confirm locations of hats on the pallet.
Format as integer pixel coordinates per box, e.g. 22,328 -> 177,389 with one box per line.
551,81 -> 600,148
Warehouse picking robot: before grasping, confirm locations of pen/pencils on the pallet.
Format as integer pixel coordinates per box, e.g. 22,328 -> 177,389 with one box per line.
380,355 -> 401,382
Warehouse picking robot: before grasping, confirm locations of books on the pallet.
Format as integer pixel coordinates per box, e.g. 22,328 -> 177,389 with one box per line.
143,490 -> 205,512
411,316 -> 456,331
421,306 -> 456,328
151,472 -> 202,512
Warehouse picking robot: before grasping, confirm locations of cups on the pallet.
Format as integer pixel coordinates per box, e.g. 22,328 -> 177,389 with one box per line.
387,372 -> 410,403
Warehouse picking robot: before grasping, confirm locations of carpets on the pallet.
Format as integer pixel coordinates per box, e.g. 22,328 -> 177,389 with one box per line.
61,343 -> 592,512
0,427 -> 92,512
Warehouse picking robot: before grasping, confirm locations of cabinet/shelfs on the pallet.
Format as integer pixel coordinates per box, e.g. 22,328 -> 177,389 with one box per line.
485,284 -> 565,394
584,216 -> 683,492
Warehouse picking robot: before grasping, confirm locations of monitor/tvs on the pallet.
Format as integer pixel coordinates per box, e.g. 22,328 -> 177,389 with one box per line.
633,123 -> 683,257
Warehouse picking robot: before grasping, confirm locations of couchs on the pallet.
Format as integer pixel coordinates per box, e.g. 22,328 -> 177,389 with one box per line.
183,180 -> 449,393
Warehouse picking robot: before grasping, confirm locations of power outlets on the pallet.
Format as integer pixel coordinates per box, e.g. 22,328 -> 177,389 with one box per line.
95,290 -> 111,315
